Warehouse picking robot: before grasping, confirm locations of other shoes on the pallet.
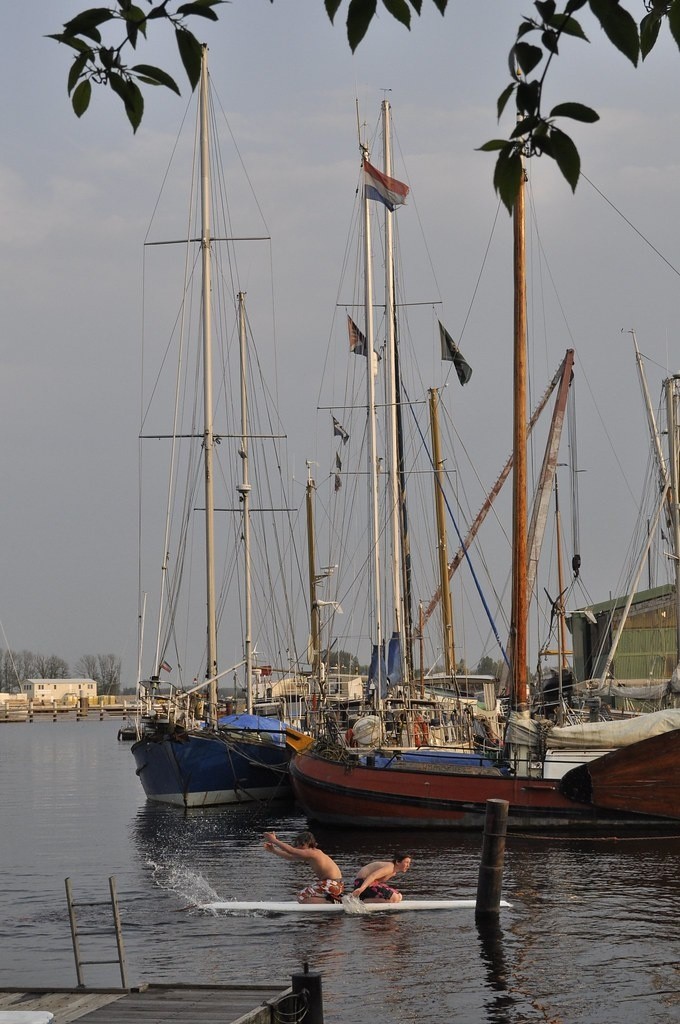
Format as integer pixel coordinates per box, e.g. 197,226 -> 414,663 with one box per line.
327,894 -> 344,904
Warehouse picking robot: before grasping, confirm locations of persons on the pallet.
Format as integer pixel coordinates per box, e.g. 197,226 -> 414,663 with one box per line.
352,852 -> 411,902
263,831 -> 345,904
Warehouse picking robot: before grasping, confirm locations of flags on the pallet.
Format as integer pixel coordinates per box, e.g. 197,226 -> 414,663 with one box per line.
347,315 -> 382,361
364,159 -> 410,213
335,453 -> 342,472
438,320 -> 473,386
333,417 -> 349,445
335,474 -> 342,491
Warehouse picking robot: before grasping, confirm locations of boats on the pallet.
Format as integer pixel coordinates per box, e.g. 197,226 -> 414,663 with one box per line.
286,702 -> 680,832
130,713 -> 299,806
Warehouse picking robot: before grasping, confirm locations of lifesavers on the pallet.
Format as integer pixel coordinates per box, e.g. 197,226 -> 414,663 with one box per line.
413,715 -> 429,747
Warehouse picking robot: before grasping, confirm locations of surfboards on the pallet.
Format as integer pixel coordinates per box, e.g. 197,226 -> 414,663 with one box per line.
197,898 -> 514,912
